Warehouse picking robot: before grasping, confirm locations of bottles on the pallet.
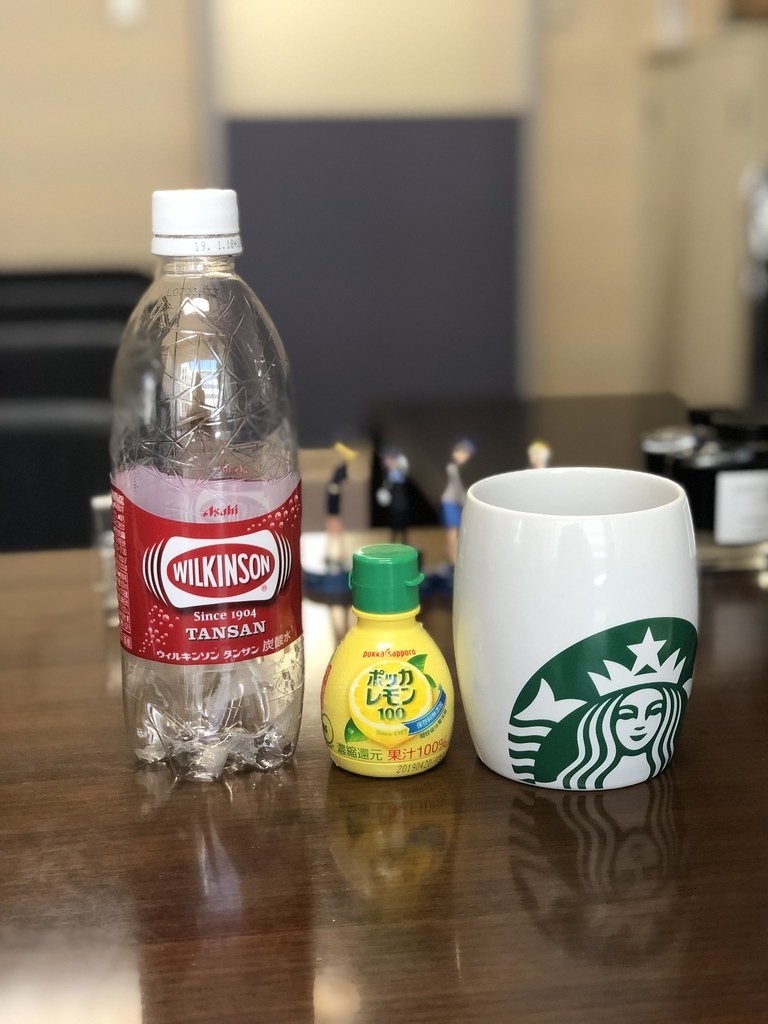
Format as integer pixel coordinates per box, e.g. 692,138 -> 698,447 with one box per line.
108,189 -> 304,779
320,543 -> 454,778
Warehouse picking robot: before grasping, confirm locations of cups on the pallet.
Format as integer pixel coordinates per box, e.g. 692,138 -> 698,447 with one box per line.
453,467 -> 698,789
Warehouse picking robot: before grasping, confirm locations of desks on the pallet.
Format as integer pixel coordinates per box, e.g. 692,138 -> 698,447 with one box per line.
0,527 -> 768,1023
